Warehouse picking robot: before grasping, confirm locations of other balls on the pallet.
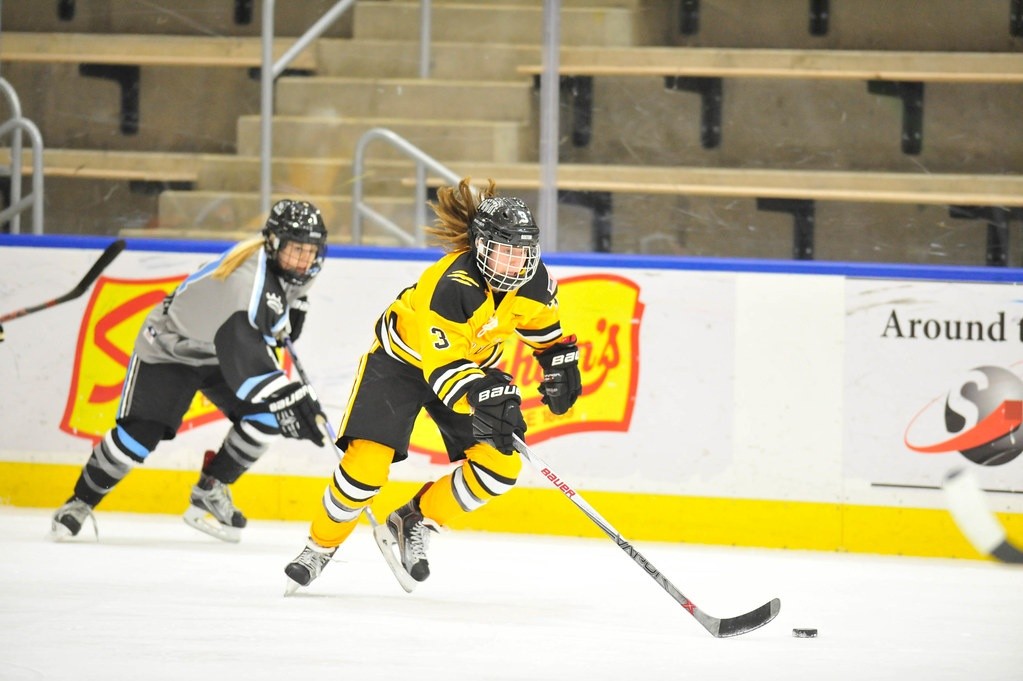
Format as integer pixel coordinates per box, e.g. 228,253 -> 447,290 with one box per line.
793,627 -> 818,635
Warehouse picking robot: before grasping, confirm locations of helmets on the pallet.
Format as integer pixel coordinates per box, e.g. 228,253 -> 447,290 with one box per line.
469,197 -> 541,291
263,199 -> 327,287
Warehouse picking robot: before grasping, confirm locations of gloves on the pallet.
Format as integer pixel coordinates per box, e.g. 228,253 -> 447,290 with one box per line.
265,381 -> 327,448
533,334 -> 582,415
276,295 -> 308,347
466,367 -> 527,455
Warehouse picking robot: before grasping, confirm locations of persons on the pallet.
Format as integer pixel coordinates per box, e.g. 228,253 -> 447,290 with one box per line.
51,199 -> 326,545
284,177 -> 583,595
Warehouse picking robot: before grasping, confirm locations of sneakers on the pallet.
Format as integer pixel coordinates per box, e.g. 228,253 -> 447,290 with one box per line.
373,482 -> 443,593
284,535 -> 338,598
50,494 -> 98,542
184,450 -> 247,543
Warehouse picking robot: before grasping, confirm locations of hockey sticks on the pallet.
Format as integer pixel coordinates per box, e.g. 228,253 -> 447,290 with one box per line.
936,468 -> 1022,564
1,239 -> 129,328
286,337 -> 456,593
512,428 -> 779,636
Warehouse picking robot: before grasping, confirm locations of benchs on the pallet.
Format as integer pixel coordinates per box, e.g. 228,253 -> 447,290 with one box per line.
519,42 -> 1023,155
404,161 -> 1023,267
1,145 -> 205,230
0,31 -> 317,133
